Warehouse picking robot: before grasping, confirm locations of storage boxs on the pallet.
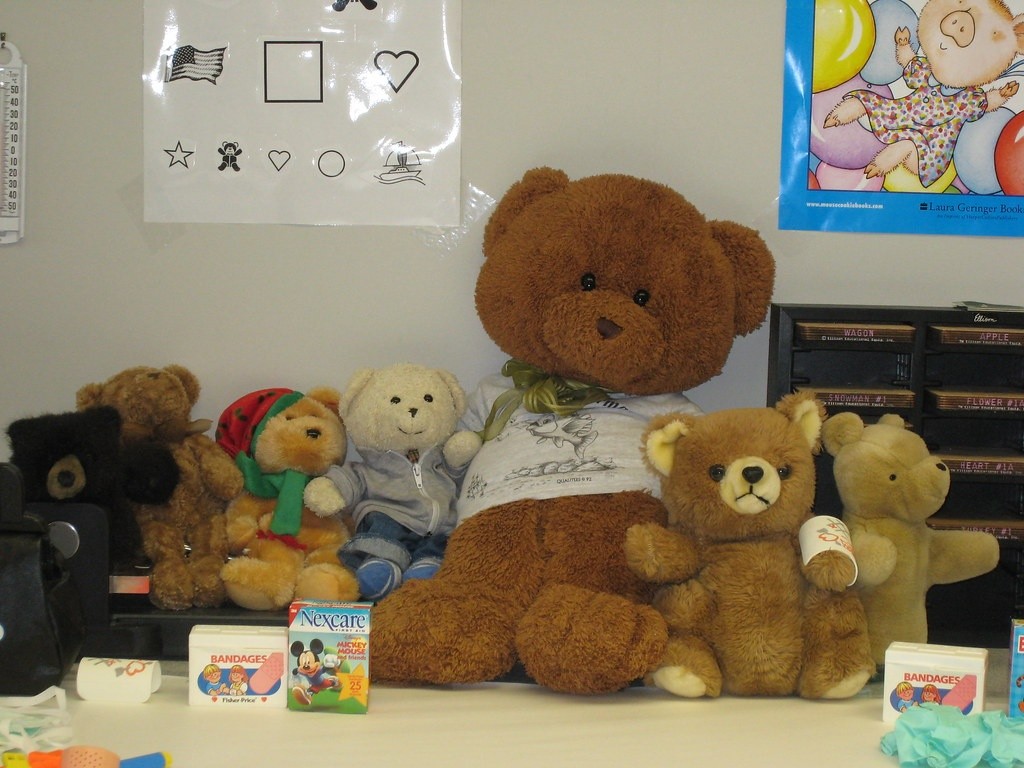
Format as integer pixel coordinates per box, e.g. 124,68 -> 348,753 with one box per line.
289,597 -> 375,716
188,624 -> 292,710
883,639 -> 991,733
1006,618 -> 1024,718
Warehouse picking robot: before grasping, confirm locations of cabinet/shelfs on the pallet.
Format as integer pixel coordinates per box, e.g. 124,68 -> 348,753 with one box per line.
769,303 -> 1024,637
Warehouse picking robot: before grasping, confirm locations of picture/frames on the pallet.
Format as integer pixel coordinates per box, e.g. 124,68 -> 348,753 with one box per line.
776,0 -> 1024,238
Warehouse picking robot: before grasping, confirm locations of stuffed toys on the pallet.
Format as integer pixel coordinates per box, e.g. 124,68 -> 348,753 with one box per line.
368,166 -> 772,692
0,365 -> 481,613
621,391 -> 1000,698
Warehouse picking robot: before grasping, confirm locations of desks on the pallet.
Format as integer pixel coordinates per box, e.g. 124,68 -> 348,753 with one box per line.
0,649 -> 1024,768
